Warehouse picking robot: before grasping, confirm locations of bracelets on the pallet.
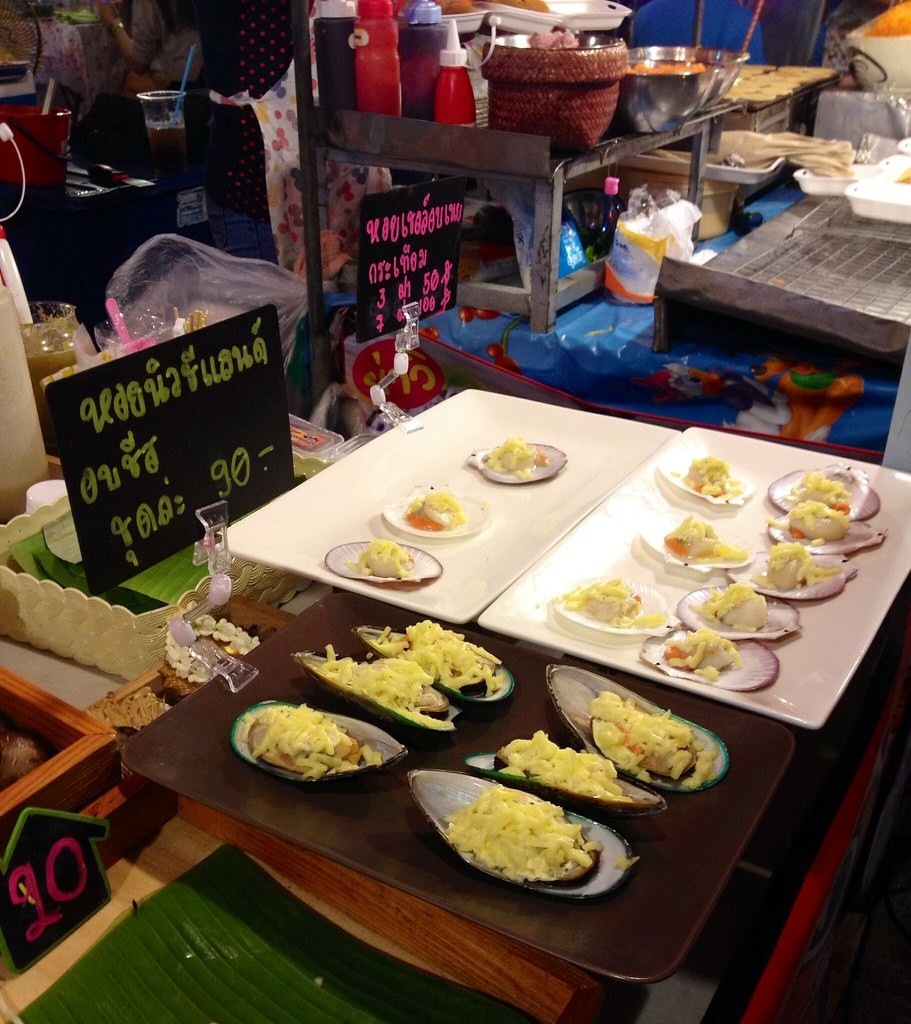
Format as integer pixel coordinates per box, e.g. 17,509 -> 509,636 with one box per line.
108,22 -> 124,36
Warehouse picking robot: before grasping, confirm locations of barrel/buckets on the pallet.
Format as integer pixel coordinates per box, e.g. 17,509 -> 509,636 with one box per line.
621,169 -> 740,241
0,105 -> 71,186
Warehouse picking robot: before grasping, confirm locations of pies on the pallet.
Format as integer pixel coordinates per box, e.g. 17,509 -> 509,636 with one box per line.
724,65 -> 838,101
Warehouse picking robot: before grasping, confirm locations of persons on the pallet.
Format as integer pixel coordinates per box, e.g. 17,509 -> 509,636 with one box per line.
97,0 -> 393,275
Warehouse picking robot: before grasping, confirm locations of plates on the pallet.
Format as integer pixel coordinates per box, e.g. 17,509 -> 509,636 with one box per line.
478,424 -> 911,730
225,390 -> 683,624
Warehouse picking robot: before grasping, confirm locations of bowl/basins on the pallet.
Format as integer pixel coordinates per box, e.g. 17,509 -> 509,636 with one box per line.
440,0 -> 751,154
793,136 -> 911,224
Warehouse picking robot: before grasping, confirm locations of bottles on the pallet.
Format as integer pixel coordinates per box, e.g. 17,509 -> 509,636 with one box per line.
0,221 -> 50,522
435,18 -> 476,127
314,0 -> 356,111
353,0 -> 400,118
398,0 -> 446,122
590,177 -> 624,256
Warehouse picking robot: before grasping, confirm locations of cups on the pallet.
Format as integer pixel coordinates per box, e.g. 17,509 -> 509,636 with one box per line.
20,301 -> 81,457
136,91 -> 188,178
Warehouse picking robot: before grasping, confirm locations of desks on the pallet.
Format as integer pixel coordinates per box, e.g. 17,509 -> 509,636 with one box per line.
41,22 -> 127,116
0,154 -> 213,351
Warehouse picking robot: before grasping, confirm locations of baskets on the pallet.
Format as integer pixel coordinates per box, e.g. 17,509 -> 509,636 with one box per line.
482,32 -> 629,152
